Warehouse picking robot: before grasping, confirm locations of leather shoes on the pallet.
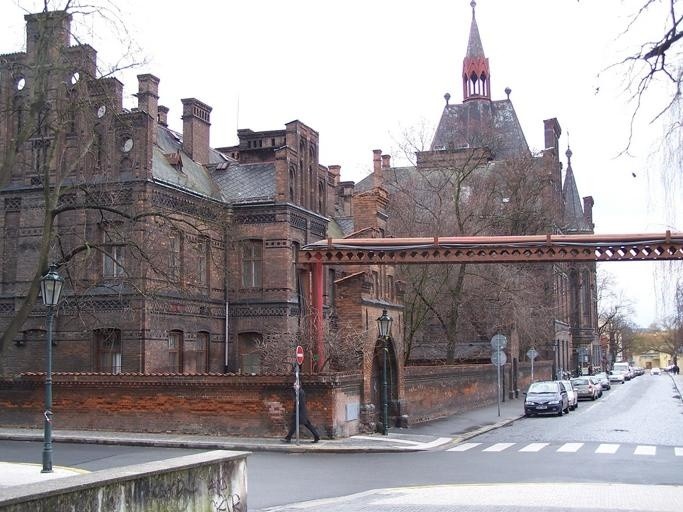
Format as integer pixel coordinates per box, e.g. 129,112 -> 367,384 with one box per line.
311,437 -> 321,443
280,438 -> 291,443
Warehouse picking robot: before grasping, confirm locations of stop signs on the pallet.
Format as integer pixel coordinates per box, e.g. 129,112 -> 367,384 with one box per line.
296,344 -> 305,366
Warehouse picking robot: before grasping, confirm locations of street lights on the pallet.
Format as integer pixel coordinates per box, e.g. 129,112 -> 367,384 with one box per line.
378,307 -> 393,438
39,261 -> 65,472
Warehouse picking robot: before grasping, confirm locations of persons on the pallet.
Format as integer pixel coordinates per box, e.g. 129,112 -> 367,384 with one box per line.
279,380 -> 321,443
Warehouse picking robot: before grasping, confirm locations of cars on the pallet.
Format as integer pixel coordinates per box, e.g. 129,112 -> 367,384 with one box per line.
524,362 -> 646,418
650,365 -> 679,376
